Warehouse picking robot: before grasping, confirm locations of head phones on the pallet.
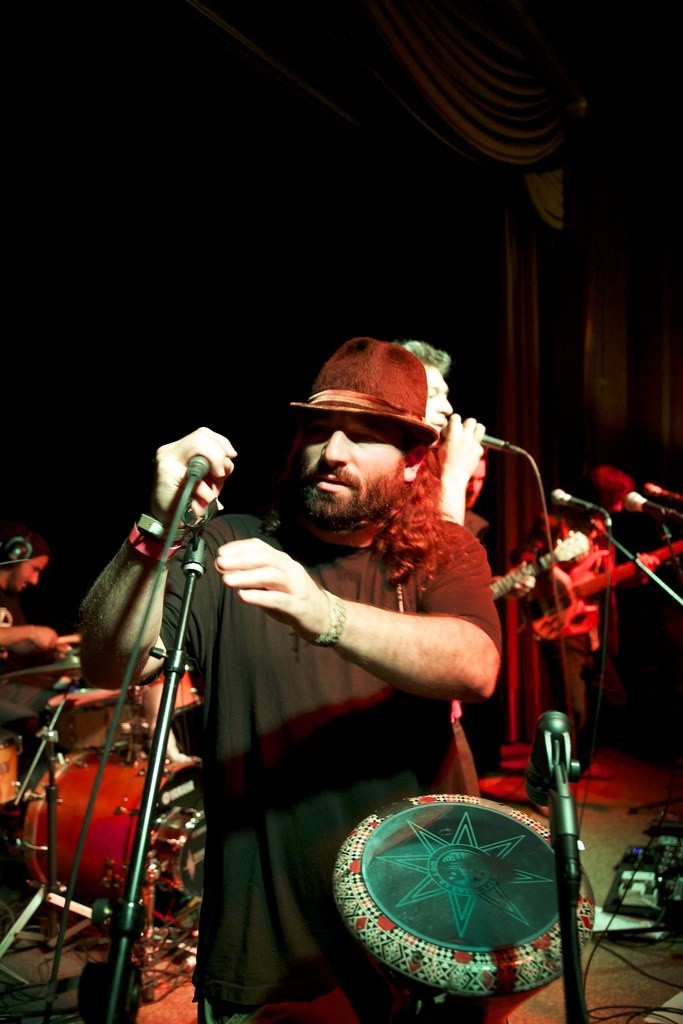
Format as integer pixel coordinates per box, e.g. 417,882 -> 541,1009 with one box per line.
4,531 -> 35,568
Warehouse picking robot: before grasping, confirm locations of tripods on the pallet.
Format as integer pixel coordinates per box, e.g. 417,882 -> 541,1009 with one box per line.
0,669 -> 204,1001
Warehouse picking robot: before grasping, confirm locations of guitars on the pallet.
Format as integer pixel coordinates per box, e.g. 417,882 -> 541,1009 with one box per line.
491,529 -> 592,601
534,539 -> 683,644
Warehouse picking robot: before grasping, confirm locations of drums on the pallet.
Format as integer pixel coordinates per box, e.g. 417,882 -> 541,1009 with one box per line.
18,744 -> 207,937
44,667 -> 203,748
1,727 -> 23,805
331,794 -> 599,1023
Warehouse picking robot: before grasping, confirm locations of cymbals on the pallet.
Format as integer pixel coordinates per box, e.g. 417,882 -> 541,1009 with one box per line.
1,655 -> 88,681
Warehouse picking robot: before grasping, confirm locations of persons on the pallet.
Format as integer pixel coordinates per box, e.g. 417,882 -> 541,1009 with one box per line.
0,528 -> 70,726
464,444 -> 536,600
515,468 -> 661,779
79,341 -> 506,1024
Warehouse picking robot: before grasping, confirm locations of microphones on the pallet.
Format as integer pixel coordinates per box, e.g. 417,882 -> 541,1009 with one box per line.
188,455 -> 211,479
442,423 -> 527,456
623,493 -> 683,521
642,483 -> 683,503
550,489 -> 609,515
526,711 -> 582,905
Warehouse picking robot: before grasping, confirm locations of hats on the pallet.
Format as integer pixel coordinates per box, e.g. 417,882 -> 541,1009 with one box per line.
290,337 -> 439,446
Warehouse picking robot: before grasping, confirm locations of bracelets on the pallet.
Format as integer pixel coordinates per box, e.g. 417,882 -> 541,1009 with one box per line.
301,587 -> 345,647
128,523 -> 184,561
137,515 -> 184,544
400,341 -> 484,796
